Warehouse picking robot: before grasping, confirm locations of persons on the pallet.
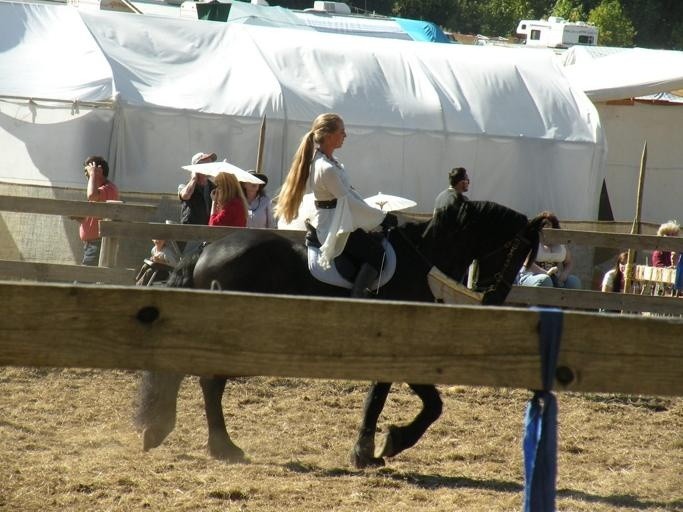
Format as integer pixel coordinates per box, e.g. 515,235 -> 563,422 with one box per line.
515,210 -> 582,289
178,151 -> 218,226
209,172 -> 249,227
271,112 -> 398,298
434,167 -> 470,210
73,155 -> 119,284
240,169 -> 274,228
652,221 -> 680,296
136,237 -> 176,287
600,251 -> 641,315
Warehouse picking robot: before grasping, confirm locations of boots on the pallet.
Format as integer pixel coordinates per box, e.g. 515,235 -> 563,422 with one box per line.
352,264 -> 377,298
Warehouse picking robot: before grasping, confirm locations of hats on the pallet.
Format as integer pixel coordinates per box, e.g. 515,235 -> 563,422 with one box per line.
239,170 -> 268,188
192,152 -> 217,165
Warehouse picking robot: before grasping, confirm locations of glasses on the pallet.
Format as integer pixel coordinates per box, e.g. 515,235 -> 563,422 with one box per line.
463,179 -> 470,184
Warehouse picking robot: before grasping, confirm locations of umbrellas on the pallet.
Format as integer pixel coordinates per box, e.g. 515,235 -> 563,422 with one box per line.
363,191 -> 418,213
182,157 -> 266,186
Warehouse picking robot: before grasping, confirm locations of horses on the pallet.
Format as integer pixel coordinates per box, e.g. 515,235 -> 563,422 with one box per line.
130,200 -> 549,469
